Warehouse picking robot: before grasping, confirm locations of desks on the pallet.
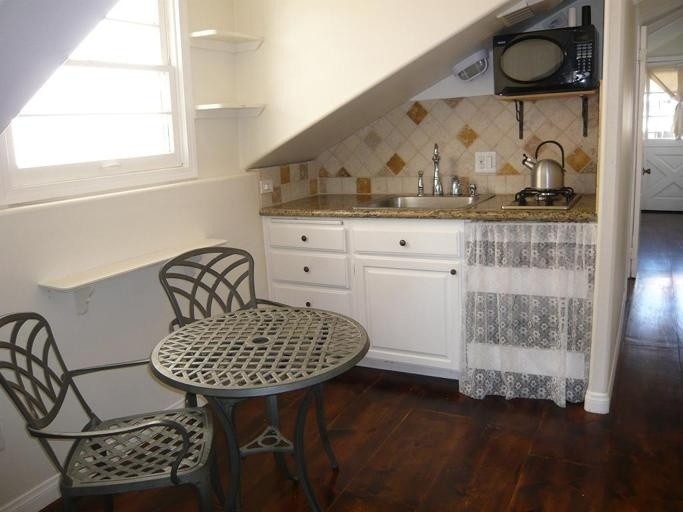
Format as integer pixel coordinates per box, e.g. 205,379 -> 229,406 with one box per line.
150,307 -> 370,511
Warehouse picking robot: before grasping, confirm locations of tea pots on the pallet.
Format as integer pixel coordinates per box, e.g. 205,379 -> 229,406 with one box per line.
522,140 -> 565,191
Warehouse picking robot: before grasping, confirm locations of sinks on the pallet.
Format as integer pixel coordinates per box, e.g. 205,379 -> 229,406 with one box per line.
375,196 -> 481,209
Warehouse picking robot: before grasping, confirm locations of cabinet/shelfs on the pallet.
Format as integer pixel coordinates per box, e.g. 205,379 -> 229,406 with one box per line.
190,28 -> 267,119
464,219 -> 594,380
351,217 -> 462,380
263,218 -> 351,316
493,90 -> 597,139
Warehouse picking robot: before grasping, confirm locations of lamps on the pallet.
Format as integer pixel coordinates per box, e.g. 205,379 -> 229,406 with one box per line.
452,49 -> 489,82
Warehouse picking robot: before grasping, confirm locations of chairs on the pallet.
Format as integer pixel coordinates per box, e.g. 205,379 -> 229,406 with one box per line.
159,247 -> 340,472
0,312 -> 224,510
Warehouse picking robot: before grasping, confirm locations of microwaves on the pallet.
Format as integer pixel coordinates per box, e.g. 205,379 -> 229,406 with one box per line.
492,23 -> 599,97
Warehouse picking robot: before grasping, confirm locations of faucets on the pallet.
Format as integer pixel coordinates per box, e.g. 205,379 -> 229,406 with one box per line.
430,143 -> 443,196
